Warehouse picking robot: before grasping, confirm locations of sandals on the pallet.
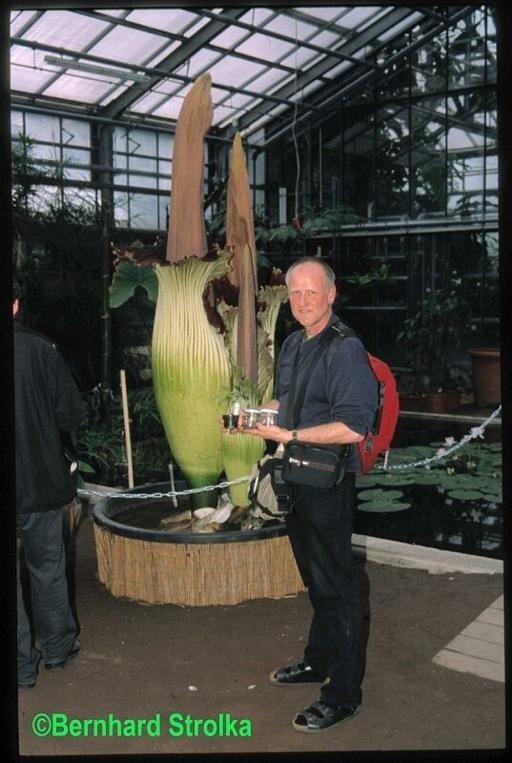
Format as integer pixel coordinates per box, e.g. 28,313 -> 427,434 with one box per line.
270,662 -> 334,687
291,683 -> 364,734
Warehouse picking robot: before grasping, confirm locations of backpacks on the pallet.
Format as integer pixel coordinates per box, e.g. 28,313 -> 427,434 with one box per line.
321,328 -> 402,477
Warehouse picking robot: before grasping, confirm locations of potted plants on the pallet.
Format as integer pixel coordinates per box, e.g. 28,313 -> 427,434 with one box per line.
94,71 -> 311,610
393,276 -> 477,413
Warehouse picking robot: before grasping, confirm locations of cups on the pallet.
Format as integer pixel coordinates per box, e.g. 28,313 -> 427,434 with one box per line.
220,402 -> 241,430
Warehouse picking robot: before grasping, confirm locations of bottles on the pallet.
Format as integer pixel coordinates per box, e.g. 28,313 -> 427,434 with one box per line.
244,408 -> 281,430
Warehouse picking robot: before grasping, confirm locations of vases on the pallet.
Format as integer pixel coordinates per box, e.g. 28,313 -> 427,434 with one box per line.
466,347 -> 502,409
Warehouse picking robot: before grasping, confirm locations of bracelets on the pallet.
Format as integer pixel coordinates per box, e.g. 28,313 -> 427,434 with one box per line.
293,430 -> 297,441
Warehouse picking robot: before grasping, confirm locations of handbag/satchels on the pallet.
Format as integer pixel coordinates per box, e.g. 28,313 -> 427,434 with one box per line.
282,439 -> 350,492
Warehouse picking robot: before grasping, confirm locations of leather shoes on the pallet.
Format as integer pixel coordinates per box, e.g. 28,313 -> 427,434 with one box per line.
19,676 -> 40,692
43,641 -> 83,674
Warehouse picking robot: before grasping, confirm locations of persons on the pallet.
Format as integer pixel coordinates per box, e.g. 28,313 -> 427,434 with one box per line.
221,256 -> 381,733
13,280 -> 85,688
